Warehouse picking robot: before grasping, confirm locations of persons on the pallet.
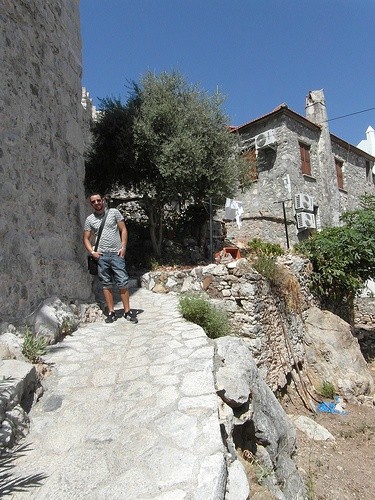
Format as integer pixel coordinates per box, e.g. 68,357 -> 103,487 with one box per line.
83,189 -> 140,325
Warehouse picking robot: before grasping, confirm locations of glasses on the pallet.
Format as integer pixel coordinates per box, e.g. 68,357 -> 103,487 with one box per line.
91,199 -> 102,204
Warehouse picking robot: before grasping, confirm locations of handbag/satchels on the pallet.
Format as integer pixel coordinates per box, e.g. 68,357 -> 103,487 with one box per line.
88,254 -> 98,276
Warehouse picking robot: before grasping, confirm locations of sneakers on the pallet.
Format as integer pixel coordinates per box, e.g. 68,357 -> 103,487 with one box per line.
105,311 -> 118,323
122,309 -> 138,324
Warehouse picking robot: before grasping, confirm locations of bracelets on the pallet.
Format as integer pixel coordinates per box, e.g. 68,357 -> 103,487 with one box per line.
91,251 -> 95,255
